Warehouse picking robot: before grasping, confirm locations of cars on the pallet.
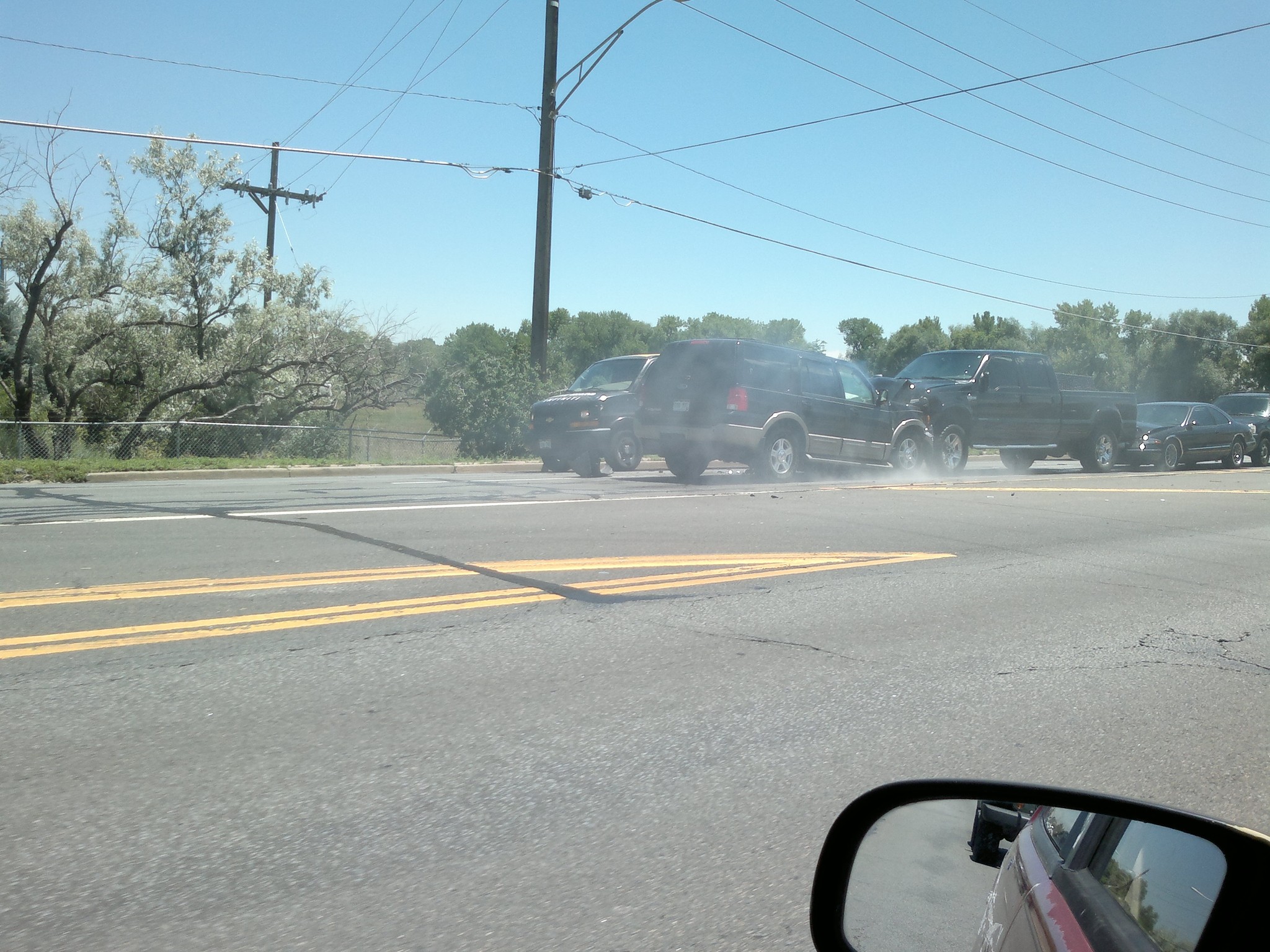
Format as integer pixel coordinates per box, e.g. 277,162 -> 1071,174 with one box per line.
1116,401 -> 1256,473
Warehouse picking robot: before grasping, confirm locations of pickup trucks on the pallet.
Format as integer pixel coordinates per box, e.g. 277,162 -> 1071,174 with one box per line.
877,348 -> 1136,476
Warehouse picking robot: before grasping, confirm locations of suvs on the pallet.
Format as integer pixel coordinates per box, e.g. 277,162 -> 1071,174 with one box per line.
525,352 -> 660,474
1211,389 -> 1269,467
634,340 -> 929,480
